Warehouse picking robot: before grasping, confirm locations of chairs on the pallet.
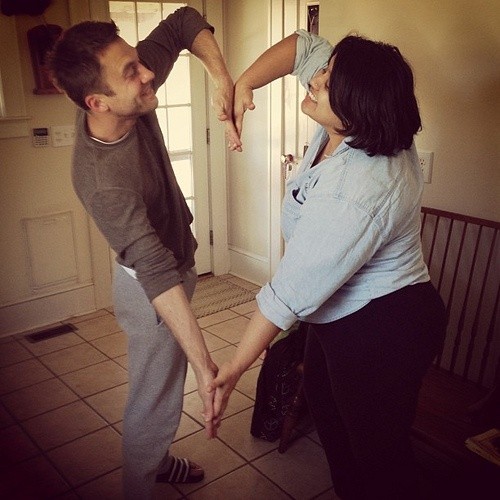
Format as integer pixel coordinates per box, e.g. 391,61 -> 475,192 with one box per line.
404,207 -> 500,481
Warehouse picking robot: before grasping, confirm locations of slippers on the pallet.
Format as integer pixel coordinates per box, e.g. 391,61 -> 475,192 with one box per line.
155,455 -> 205,485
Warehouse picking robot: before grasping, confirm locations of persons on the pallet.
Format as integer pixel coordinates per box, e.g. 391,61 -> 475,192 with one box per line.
48,5 -> 242,500
207,32 -> 448,500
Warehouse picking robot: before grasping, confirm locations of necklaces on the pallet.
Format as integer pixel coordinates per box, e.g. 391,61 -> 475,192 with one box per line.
323,154 -> 333,158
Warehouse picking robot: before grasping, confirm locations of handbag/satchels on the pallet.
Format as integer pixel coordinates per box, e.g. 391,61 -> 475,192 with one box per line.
250,330 -> 305,443
